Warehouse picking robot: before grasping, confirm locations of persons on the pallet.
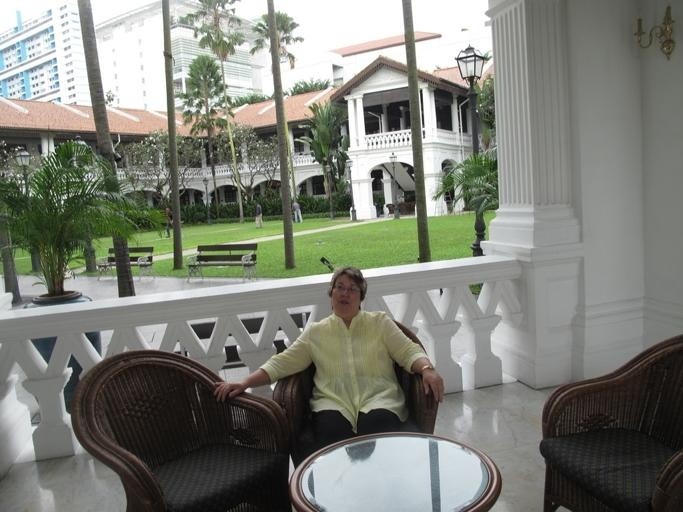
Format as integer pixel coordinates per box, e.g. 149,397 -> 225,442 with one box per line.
291,201 -> 304,223
164,206 -> 177,238
252,201 -> 264,230
213,264 -> 444,449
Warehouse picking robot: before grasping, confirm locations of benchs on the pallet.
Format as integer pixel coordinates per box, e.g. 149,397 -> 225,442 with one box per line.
185,244 -> 258,283
96,247 -> 154,281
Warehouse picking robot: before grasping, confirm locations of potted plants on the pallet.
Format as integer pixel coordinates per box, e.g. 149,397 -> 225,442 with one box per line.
0,140 -> 169,308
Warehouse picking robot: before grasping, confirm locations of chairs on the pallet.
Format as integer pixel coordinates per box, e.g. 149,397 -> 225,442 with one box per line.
273,320 -> 439,468
72,349 -> 291,511
539,334 -> 682,512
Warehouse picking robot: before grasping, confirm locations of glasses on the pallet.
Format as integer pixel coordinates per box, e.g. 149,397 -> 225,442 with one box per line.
332,284 -> 361,295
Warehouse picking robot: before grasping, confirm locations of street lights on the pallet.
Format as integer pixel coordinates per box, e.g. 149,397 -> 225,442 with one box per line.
15,148 -> 43,272
203,153 -> 400,225
457,44 -> 489,255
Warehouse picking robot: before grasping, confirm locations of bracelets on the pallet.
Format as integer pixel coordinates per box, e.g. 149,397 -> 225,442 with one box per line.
421,364 -> 437,373
